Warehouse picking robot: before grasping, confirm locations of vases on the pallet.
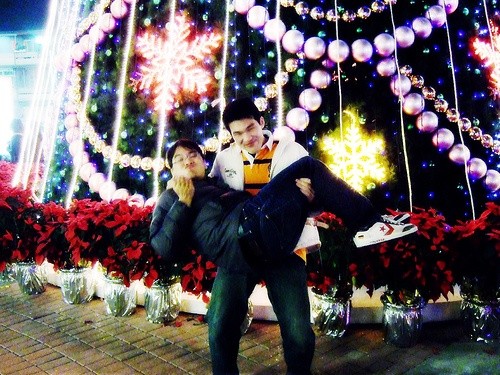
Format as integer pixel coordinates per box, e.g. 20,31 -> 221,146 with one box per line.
310,286 -> 353,341
458,288 -> 500,355
98,272 -> 138,317
144,282 -> 181,326
379,293 -> 426,348
58,262 -> 95,305
241,297 -> 254,336
15,259 -> 47,295
0,262 -> 17,290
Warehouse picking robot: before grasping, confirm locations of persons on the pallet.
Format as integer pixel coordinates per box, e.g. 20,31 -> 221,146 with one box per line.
150,140 -> 417,274
207,98 -> 321,375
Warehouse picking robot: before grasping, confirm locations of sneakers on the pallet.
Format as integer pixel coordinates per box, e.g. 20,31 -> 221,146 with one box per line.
353,216 -> 418,247
383,212 -> 410,221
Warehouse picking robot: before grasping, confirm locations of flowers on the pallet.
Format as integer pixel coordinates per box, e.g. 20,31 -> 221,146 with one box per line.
0,159 -> 500,343
